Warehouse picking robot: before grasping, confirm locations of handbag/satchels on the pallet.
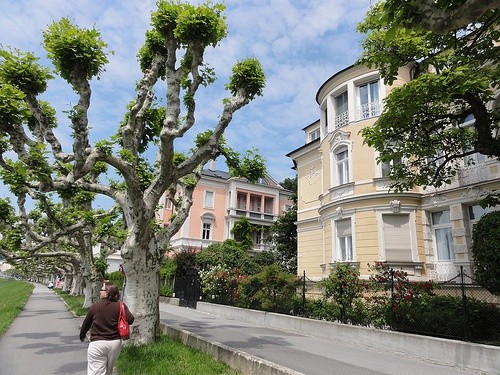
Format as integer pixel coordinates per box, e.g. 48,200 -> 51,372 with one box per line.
118,302 -> 130,340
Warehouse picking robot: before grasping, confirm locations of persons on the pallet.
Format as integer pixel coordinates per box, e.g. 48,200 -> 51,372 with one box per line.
80,282 -> 134,375
48,282 -> 53,288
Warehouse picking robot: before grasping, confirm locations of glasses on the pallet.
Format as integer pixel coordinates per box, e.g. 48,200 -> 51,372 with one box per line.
98,288 -> 106,291
